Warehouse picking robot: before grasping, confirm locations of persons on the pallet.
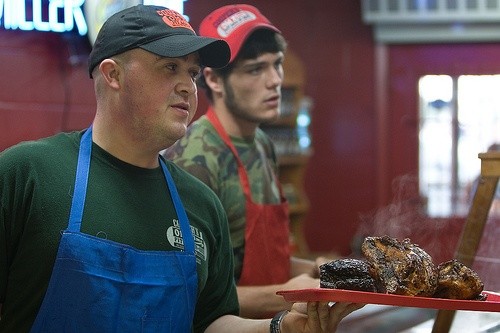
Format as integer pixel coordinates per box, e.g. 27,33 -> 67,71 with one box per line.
164,5 -> 336,316
0,4 -> 367,333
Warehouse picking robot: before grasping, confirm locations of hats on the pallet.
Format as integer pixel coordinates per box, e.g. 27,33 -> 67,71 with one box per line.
88,3 -> 231,79
199,3 -> 282,69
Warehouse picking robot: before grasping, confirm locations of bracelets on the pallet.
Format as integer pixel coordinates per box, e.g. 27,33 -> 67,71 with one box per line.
269,310 -> 288,333
